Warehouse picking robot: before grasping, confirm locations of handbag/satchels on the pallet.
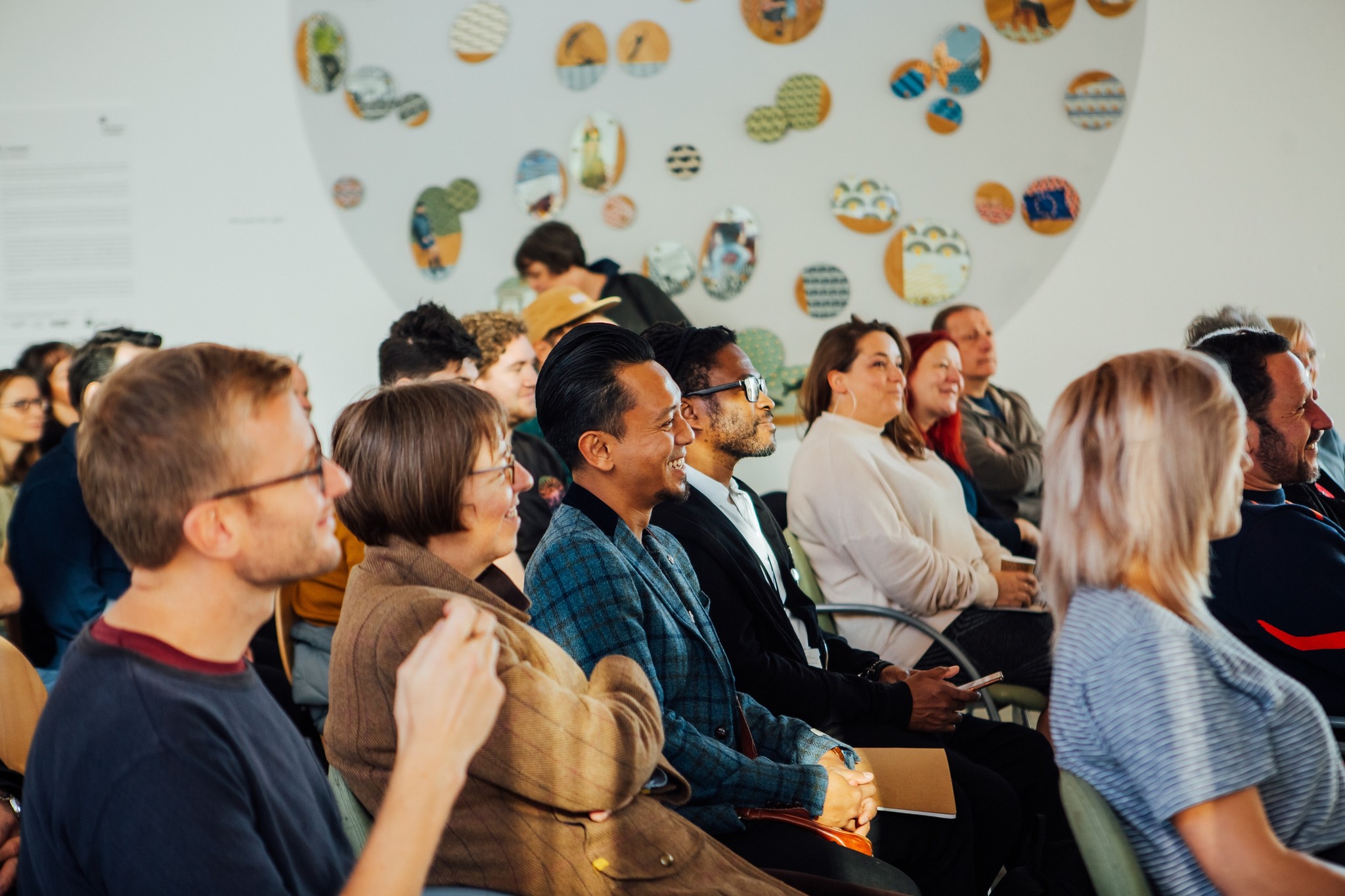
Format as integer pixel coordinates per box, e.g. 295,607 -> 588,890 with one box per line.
741,801 -> 873,857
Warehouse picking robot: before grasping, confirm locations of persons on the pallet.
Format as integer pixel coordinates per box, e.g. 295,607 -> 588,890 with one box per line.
2,221 -> 1344,896
320,377 -> 806,894
14,340 -> 507,894
412,202 -> 458,276
309,15 -> 344,92
1036,347 -> 1343,894
710,206 -> 752,278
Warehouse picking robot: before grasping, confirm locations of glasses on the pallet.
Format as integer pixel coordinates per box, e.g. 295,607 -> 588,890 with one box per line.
0,398 -> 47,414
683,378 -> 768,403
212,425 -> 326,498
466,453 -> 516,484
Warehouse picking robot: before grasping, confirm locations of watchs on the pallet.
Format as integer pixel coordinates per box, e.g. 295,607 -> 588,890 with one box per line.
1,790 -> 21,821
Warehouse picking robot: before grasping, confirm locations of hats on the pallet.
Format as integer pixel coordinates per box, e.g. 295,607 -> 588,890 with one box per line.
522,285 -> 622,342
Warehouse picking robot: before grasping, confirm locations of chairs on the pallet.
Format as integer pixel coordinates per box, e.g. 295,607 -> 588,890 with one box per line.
0,633 -> 51,795
275,584 -> 301,689
784,525 -> 1050,726
1057,766 -> 1152,896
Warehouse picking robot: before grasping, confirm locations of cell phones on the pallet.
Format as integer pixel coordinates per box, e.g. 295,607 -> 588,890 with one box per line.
957,671 -> 1004,691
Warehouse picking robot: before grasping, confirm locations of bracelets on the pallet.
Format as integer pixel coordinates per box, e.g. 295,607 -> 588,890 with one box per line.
833,746 -> 845,764
865,659 -> 892,681
